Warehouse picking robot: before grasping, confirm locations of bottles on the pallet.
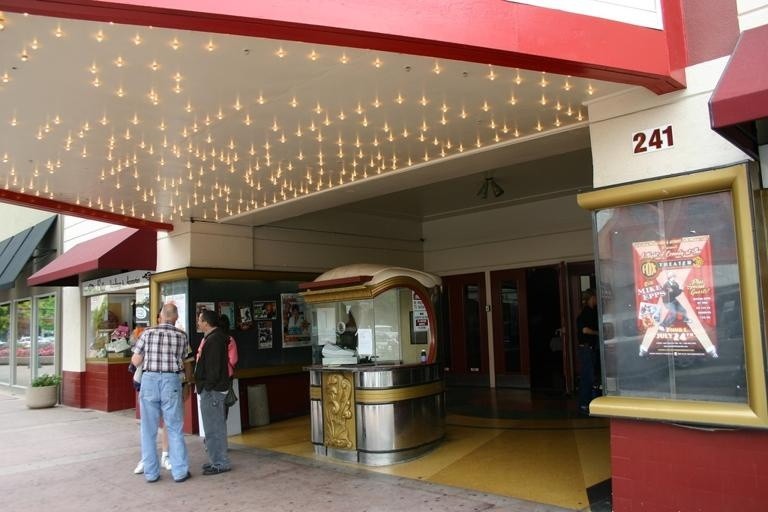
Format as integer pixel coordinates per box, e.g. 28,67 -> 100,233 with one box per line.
420,349 -> 427,363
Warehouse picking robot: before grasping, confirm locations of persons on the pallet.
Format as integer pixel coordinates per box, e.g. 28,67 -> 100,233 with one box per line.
244,309 -> 250,321
195,314 -> 239,452
131,304 -> 191,482
134,309 -> 195,474
287,304 -> 306,335
195,310 -> 231,475
575,289 -> 599,414
662,271 -> 693,325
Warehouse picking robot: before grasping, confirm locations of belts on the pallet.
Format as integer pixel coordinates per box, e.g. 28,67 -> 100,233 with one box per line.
142,370 -> 180,374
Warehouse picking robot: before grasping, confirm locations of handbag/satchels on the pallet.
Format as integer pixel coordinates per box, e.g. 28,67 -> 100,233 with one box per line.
225,389 -> 238,406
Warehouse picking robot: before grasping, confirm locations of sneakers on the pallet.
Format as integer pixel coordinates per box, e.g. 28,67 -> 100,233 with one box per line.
161,457 -> 171,470
134,461 -> 144,474
200,463 -> 229,475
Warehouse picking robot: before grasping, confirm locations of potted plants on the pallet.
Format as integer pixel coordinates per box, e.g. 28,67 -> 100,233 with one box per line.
24,374 -> 63,409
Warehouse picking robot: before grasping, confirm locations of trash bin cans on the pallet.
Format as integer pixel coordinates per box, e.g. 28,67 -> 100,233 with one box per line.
247,384 -> 270,426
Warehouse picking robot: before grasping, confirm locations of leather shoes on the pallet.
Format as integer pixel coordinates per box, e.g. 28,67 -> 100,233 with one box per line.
149,475 -> 160,483
176,472 -> 191,482
